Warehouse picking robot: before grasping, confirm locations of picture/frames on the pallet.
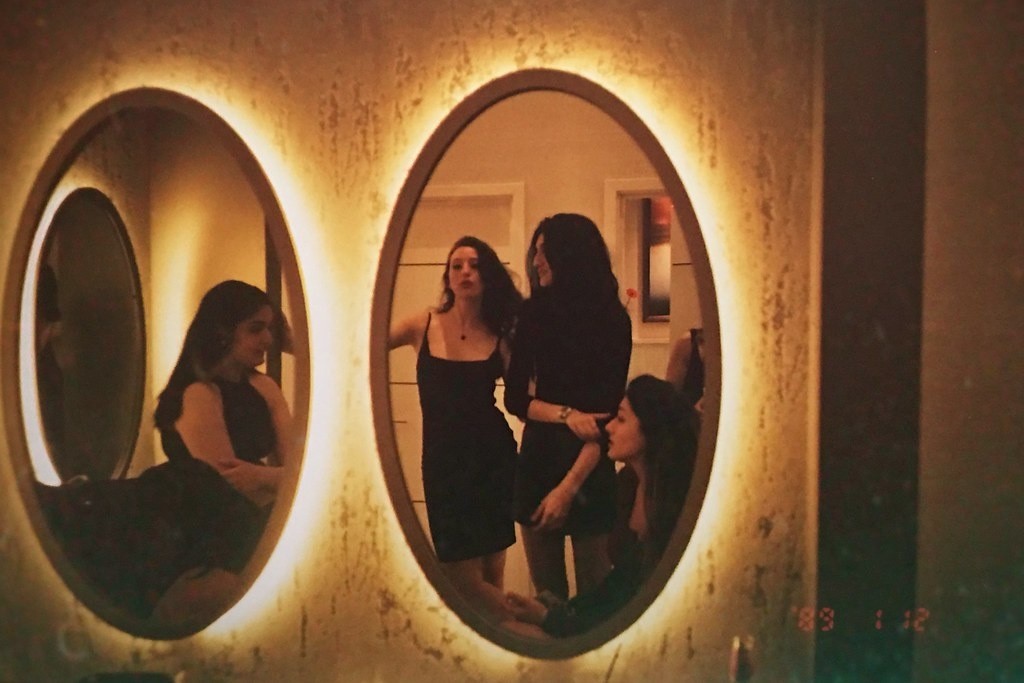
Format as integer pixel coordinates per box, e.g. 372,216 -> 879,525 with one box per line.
640,198 -> 674,323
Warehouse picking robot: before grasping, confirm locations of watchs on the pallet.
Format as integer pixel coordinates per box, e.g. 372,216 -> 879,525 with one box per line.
557,405 -> 573,420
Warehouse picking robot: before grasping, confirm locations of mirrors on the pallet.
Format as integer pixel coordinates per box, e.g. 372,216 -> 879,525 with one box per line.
34,185 -> 146,484
366,71 -> 724,662
1,88 -> 313,641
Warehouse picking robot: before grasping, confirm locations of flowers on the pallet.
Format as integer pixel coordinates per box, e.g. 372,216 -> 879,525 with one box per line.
624,288 -> 637,307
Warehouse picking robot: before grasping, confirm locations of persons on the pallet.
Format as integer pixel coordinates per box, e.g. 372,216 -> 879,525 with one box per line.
154,280 -> 292,570
388,213 -> 707,637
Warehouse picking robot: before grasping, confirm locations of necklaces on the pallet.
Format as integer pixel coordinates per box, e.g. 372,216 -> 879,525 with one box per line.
454,312 -> 478,340
